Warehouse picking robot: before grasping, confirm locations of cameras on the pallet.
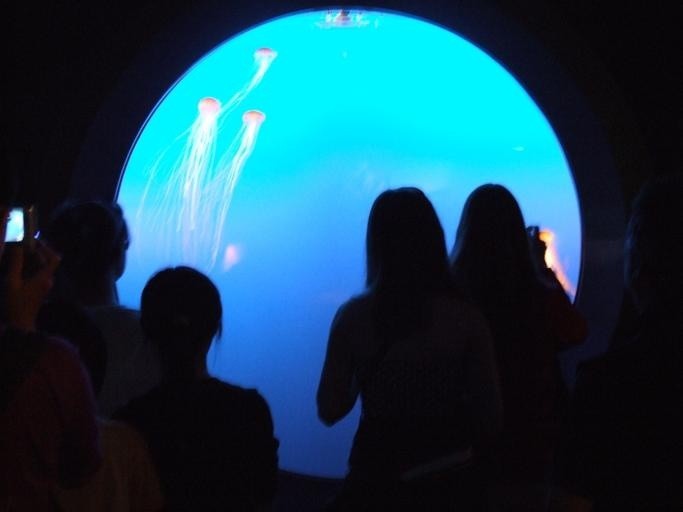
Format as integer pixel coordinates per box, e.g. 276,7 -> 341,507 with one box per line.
1,203 -> 40,256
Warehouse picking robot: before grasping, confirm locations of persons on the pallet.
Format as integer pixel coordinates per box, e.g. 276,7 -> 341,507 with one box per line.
445,183 -> 606,512
39,189 -> 209,422
552,178 -> 681,512
107,263 -> 282,512
312,184 -> 505,511
1,163 -> 107,510
96,413 -> 166,512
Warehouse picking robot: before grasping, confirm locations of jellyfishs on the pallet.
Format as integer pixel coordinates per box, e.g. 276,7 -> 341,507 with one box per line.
133,97 -> 221,272
201,109 -> 266,277
215,46 -> 279,121
540,228 -> 576,296
